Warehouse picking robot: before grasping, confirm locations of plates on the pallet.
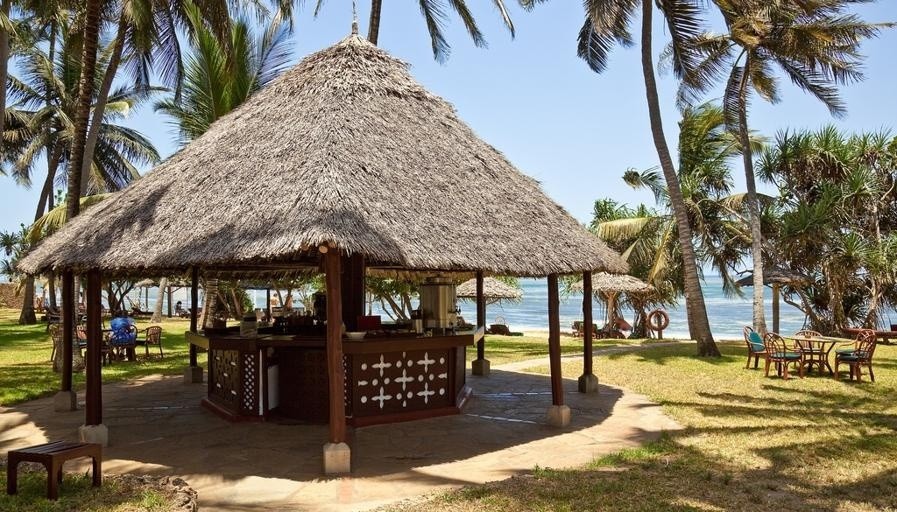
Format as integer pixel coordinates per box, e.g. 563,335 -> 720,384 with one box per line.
344,331 -> 367,340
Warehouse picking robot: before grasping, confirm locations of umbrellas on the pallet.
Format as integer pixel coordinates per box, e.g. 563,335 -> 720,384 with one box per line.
735,264 -> 811,338
456,277 -> 522,331
572,271 -> 656,338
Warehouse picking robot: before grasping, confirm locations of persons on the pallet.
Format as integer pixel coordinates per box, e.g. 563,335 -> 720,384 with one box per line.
174,300 -> 185,315
122,310 -> 135,334
110,309 -> 137,359
270,293 -> 279,313
284,294 -> 294,310
80,317 -> 89,341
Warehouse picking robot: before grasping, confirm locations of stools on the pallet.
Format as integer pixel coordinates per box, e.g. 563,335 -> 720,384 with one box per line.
8,439 -> 102,501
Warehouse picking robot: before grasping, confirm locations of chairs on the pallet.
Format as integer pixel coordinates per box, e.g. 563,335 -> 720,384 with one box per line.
742,326 -> 878,384
44,303 -> 163,366
